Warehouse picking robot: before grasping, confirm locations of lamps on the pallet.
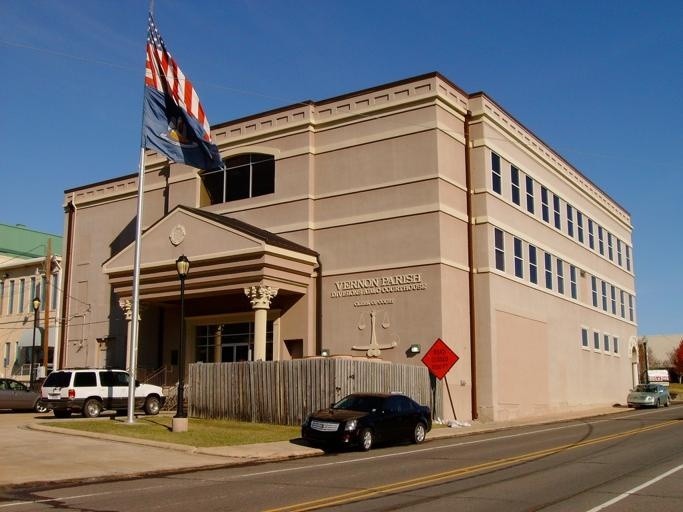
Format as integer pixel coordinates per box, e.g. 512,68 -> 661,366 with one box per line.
321,349 -> 329,357
410,345 -> 420,354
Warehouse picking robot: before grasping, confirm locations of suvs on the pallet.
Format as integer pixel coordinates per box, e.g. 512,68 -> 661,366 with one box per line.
39,367 -> 166,418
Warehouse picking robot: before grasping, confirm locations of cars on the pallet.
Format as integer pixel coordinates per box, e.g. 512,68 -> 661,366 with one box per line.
627,384 -> 670,408
301,393 -> 431,451
0,378 -> 52,413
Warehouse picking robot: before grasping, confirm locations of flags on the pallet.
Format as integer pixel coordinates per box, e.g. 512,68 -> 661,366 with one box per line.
140,10 -> 225,172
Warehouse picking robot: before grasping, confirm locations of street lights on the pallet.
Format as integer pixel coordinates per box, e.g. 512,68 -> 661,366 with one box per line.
174,253 -> 192,418
29,296 -> 40,390
